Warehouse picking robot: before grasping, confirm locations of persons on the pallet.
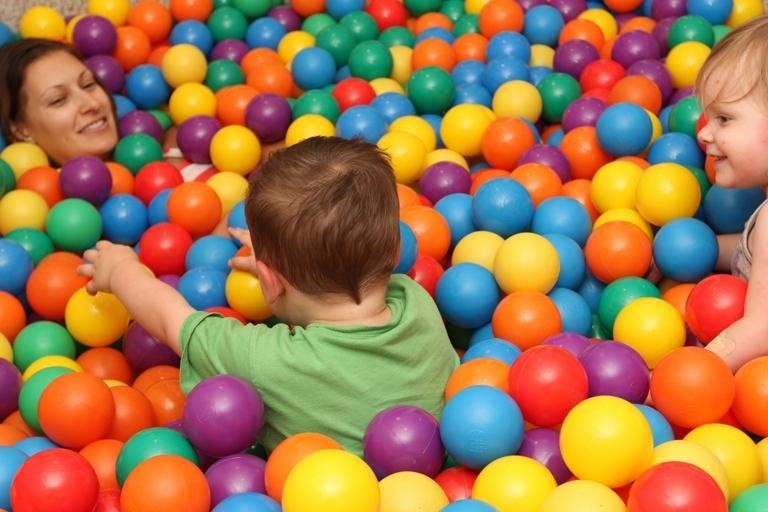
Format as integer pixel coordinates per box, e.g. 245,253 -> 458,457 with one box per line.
0,38 -> 121,168
74,132 -> 461,468
697,12 -> 768,373
